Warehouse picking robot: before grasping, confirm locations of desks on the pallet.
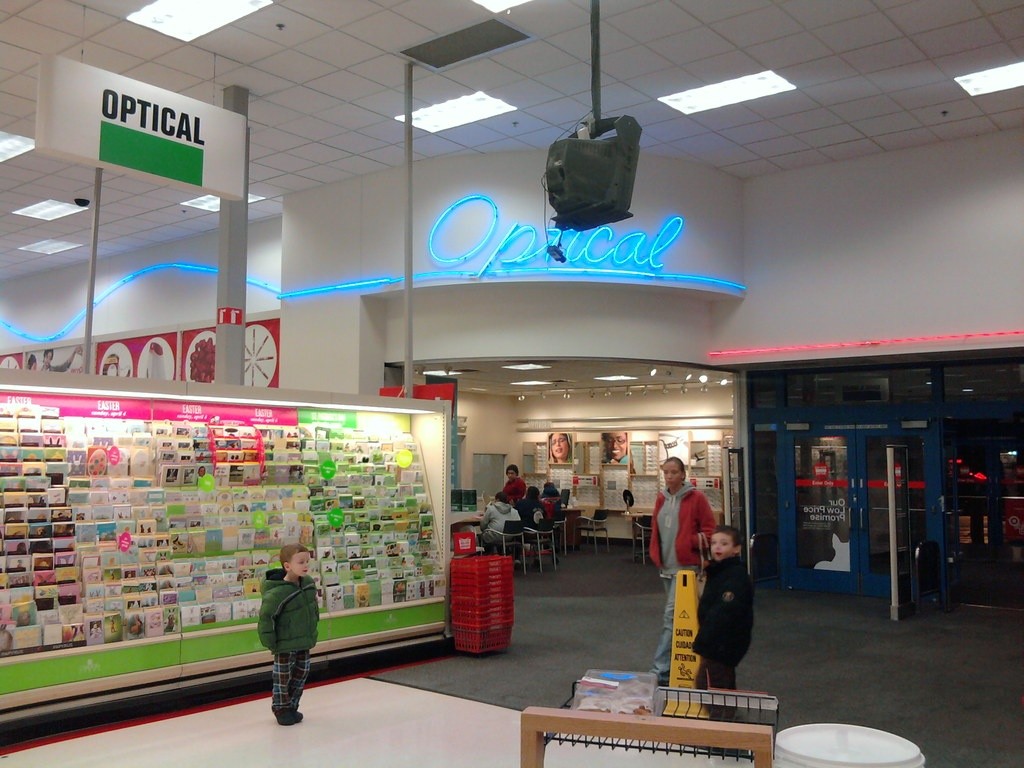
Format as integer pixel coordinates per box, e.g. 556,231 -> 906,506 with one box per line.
620,512 -> 653,566
555,509 -> 586,546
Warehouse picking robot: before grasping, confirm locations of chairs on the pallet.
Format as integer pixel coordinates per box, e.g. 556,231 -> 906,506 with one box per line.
632,520 -> 653,566
559,489 -> 569,508
572,508 -> 610,554
513,518 -> 556,574
480,520 -> 528,576
541,508 -> 567,557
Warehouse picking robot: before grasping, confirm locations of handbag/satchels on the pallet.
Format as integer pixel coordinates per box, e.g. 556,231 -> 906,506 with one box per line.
696,531 -> 712,599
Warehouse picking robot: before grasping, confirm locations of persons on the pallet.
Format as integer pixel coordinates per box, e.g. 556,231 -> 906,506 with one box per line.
28,346 -> 83,372
480,491 -> 521,556
502,464 -> 526,502
541,482 -> 561,520
648,457 -> 715,682
549,433 -> 573,463
602,433 -> 628,464
515,486 -> 546,564
692,526 -> 755,689
194,430 -> 205,481
258,543 -> 320,725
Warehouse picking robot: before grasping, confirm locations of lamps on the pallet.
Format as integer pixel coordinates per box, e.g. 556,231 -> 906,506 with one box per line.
518,365 -> 733,401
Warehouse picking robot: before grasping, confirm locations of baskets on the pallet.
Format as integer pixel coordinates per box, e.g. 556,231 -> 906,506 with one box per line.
450,554 -> 515,654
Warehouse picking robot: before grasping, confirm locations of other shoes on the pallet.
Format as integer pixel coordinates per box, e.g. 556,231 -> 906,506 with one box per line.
275,708 -> 304,725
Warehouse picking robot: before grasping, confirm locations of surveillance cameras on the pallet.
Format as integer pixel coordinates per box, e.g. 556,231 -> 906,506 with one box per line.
74,198 -> 90,206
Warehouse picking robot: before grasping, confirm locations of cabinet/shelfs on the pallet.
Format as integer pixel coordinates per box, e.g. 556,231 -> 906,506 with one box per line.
518,431 -> 737,529
1,367 -> 452,734
